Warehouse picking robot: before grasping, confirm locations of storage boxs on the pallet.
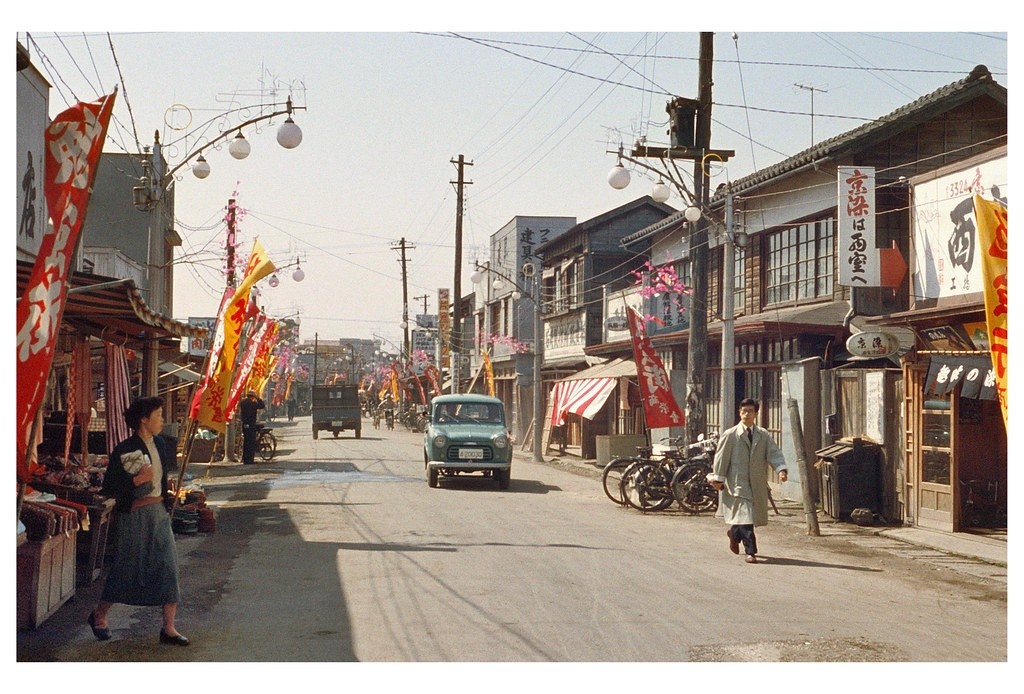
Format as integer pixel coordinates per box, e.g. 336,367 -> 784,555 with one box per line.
49,484 -> 102,505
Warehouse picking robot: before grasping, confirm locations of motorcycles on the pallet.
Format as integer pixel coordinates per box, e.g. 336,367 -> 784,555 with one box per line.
385,412 -> 395,431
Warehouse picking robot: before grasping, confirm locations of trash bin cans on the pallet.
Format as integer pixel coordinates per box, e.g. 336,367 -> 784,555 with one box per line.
815,437 -> 883,520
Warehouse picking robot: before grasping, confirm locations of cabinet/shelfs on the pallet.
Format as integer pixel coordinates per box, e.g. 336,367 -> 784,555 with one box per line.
17,494 -> 118,631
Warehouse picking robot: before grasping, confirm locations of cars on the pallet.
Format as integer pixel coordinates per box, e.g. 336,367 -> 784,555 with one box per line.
423,393 -> 514,491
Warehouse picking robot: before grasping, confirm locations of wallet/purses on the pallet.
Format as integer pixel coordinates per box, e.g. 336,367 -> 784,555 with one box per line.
120,450 -> 155,497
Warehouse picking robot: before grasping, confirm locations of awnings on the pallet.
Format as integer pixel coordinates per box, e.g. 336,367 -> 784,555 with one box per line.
548,352 -> 645,426
16,260 -> 209,341
584,304 -> 850,357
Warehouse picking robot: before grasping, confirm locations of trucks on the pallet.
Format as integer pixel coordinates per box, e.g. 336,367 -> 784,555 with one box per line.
309,385 -> 362,440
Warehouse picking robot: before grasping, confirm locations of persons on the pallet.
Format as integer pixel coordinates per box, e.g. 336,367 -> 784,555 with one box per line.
711,399 -> 788,561
378,393 -> 396,429
283,393 -> 297,422
87,397 -> 189,643
239,390 -> 265,464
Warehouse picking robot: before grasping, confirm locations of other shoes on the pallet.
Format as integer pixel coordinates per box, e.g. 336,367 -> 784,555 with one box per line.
244,459 -> 258,464
745,553 -> 756,563
727,529 -> 740,554
159,627 -> 190,646
87,611 -> 112,640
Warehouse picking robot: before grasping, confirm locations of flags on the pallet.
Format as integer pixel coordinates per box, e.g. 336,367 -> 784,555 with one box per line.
628,308 -> 685,428
974,194 -> 1008,432
190,243 -> 281,431
482,352 -> 495,397
271,376 -> 293,405
379,362 -> 444,404
15,86 -> 115,476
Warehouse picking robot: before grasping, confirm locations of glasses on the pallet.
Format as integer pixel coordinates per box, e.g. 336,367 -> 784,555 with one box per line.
739,408 -> 755,415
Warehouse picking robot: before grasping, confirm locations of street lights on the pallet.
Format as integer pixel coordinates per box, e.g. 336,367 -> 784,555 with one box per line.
471,258 -> 544,461
397,317 -> 454,396
607,143 -> 735,437
221,257 -> 310,466
141,94 -> 307,396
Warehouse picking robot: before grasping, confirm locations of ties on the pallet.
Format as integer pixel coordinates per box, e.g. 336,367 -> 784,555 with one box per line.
747,427 -> 753,444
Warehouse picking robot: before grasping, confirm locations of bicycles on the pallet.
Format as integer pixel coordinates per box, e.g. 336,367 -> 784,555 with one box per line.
395,401 -> 427,433
238,423 -> 276,461
601,431 -> 722,514
